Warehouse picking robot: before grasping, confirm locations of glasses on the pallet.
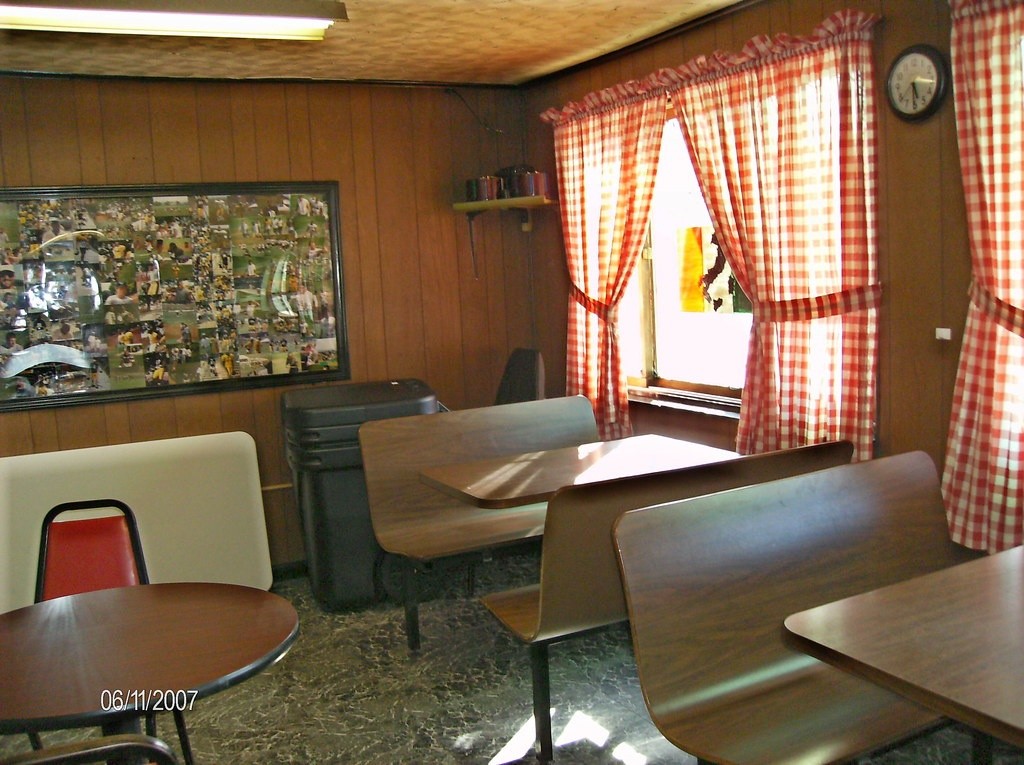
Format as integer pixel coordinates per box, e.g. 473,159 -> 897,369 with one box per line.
0,271 -> 14,278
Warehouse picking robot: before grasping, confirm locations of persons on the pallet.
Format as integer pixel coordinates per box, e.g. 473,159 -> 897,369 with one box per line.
0,194 -> 335,399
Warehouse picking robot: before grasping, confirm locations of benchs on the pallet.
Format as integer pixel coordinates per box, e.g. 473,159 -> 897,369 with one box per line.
611,450 -> 960,765
478,439 -> 854,765
358,394 -> 602,651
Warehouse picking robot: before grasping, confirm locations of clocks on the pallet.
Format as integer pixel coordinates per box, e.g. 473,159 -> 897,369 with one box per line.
884,45 -> 947,125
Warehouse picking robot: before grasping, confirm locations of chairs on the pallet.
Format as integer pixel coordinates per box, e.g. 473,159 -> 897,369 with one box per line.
25,499 -> 157,751
493,348 -> 545,406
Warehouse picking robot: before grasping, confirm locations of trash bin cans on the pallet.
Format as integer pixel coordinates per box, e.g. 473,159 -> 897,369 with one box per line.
279,378 -> 442,614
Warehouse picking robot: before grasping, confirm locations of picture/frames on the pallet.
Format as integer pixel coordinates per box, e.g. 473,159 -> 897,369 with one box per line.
0,181 -> 351,414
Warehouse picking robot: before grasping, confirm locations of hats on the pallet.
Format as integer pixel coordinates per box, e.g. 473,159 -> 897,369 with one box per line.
0,265 -> 14,271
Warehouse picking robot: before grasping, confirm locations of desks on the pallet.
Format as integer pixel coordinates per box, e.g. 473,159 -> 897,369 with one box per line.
0,582 -> 302,765
780,546 -> 1024,765
418,434 -> 742,509
